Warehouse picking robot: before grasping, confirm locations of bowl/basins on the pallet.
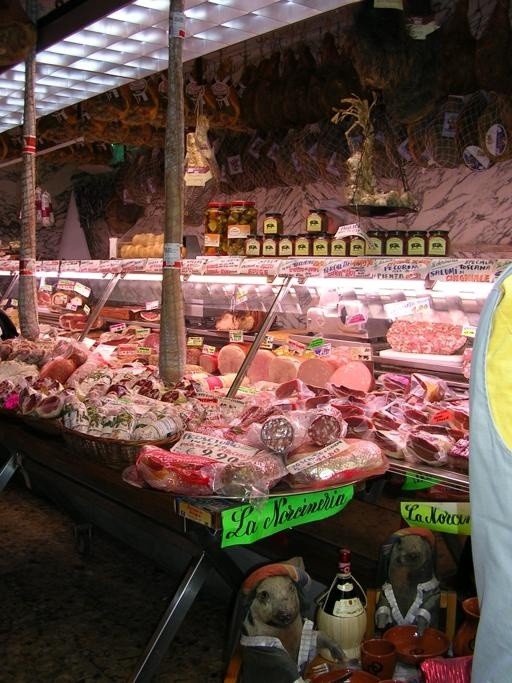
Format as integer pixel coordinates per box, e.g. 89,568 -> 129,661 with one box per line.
309,667 -> 382,682
382,623 -> 450,665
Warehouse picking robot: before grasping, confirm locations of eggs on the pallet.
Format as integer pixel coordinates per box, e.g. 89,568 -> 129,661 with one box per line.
121,234 -> 187,258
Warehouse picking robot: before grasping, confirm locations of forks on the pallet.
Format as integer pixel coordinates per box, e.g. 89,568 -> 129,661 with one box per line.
311,658 -> 360,676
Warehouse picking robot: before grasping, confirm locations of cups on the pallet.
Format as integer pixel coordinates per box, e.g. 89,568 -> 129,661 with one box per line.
358,638 -> 397,681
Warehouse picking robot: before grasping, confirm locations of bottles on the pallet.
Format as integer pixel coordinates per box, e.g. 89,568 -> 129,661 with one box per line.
452,596 -> 481,657
316,548 -> 369,663
246,209 -> 451,257
200,199 -> 257,256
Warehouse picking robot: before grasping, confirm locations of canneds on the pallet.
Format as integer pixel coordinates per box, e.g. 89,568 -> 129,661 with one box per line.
202,200 -> 450,257
